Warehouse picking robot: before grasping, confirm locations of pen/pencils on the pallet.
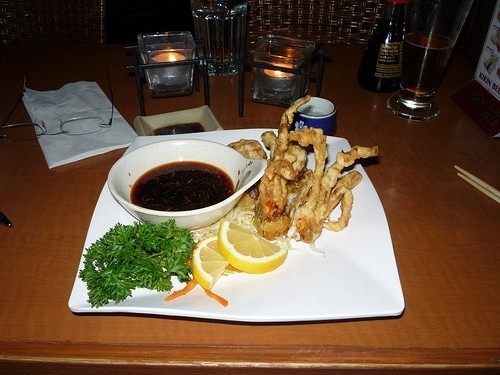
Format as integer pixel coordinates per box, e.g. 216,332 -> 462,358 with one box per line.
0,211 -> 12,227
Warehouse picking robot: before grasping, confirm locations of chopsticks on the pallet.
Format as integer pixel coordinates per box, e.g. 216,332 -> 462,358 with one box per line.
453,164 -> 500,203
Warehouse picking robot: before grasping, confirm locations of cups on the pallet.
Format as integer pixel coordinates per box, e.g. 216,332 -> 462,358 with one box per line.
385,0 -> 472,122
292,97 -> 336,136
191,0 -> 247,77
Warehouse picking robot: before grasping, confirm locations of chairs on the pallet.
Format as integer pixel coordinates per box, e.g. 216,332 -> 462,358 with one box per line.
244,0 -> 386,53
0,0 -> 106,44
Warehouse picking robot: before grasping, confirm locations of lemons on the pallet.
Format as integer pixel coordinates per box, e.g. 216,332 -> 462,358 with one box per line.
192,235 -> 230,290
218,217 -> 288,273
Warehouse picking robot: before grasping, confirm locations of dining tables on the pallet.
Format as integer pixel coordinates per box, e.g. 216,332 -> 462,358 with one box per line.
0,43 -> 500,375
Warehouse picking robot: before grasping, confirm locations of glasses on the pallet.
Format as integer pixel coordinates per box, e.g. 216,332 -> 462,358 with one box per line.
0,70 -> 113,142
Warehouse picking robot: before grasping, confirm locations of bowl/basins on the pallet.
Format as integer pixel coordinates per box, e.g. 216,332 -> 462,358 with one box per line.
108,136 -> 268,230
134,105 -> 224,137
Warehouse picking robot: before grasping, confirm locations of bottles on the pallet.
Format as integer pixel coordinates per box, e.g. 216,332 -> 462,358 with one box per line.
357,0 -> 409,93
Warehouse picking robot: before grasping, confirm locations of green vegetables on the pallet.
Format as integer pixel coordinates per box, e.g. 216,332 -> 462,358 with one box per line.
80,218 -> 199,308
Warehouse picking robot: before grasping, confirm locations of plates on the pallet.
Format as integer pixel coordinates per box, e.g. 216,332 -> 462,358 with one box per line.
67,129 -> 405,323
